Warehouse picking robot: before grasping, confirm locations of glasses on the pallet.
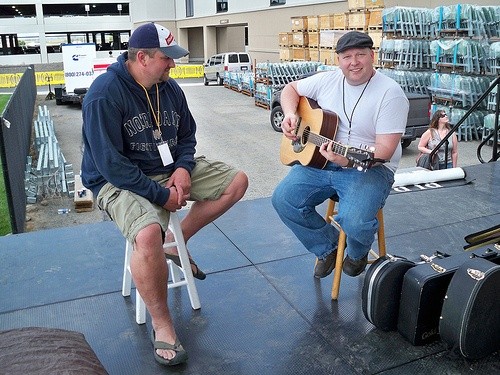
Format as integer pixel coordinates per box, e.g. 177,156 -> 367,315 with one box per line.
440,114 -> 448,118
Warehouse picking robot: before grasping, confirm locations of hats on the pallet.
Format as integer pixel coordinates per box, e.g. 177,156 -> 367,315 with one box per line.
335,31 -> 373,53
128,22 -> 190,59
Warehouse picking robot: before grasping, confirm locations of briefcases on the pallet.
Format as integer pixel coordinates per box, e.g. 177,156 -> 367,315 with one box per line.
396,243 -> 500,346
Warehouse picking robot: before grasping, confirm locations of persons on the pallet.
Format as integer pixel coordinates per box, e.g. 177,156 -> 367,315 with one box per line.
271,30 -> 410,277
81,22 -> 248,366
418,110 -> 459,171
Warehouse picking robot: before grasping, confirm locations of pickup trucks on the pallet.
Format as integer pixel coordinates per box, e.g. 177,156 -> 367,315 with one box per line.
270,71 -> 432,149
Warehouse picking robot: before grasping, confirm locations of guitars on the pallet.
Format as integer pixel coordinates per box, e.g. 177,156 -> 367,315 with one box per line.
280,96 -> 376,174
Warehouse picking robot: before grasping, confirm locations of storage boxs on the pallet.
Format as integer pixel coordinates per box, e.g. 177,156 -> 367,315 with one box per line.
278,0 -> 385,67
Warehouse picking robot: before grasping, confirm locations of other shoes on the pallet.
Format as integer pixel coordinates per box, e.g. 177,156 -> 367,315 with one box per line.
341,246 -> 370,277
313,236 -> 348,278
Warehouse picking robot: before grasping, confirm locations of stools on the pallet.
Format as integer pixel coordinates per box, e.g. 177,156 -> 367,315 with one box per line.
314,195 -> 386,300
121,211 -> 202,325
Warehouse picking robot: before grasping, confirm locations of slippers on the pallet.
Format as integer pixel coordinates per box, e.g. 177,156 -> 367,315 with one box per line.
164,253 -> 207,280
150,329 -> 188,365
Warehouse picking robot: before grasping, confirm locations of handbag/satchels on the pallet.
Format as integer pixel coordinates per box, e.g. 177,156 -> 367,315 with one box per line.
415,146 -> 435,164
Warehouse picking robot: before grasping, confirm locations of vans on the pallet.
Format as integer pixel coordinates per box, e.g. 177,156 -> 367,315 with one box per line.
202,52 -> 252,85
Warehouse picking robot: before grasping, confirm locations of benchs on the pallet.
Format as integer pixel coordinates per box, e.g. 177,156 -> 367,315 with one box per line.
74,174 -> 93,212
